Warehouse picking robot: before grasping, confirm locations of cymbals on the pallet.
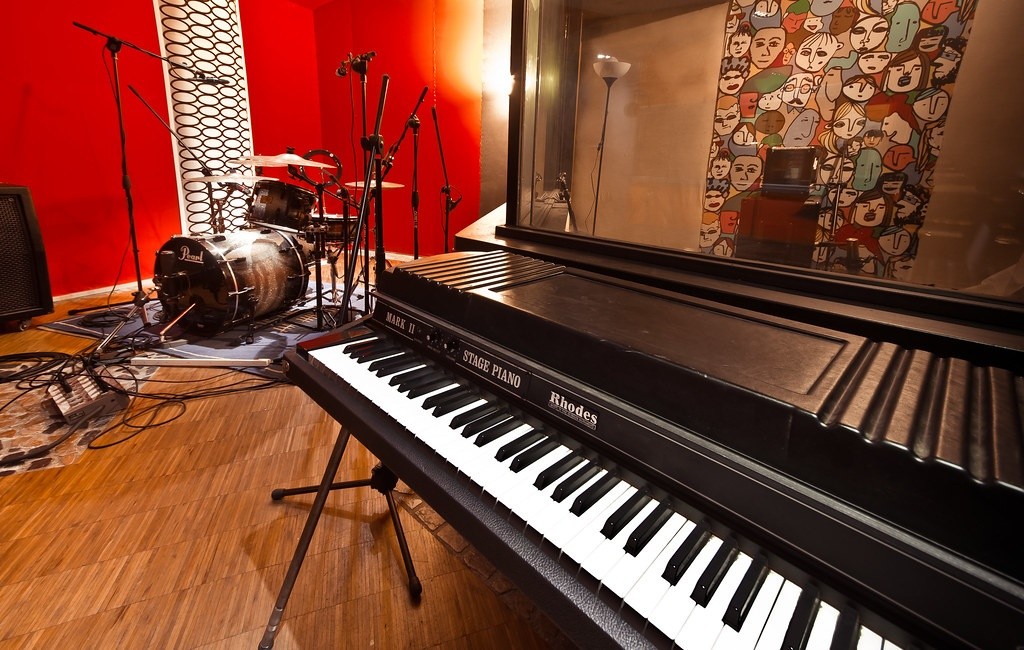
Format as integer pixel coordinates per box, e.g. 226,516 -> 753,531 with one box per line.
227,153 -> 338,169
184,173 -> 281,183
345,179 -> 405,189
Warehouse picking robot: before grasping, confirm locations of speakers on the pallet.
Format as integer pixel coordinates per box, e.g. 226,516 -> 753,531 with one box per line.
0,185 -> 54,323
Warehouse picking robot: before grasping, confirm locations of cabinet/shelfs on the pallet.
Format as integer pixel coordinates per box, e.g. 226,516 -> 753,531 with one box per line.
736,195 -> 820,268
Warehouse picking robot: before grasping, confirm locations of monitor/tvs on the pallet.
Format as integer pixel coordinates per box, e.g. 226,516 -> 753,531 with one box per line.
764,146 -> 815,190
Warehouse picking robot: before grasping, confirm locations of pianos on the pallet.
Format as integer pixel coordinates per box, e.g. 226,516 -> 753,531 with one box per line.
279,201 -> 1024,650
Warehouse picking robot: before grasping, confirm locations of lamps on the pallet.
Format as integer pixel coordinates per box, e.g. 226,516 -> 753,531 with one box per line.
593,57 -> 631,235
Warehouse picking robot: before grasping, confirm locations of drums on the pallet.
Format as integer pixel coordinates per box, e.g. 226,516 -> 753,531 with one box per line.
308,213 -> 361,244
244,180 -> 321,233
152,226 -> 311,334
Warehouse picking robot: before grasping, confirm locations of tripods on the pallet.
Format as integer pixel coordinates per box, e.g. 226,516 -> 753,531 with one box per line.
67,22 -> 206,332
228,75 -> 422,597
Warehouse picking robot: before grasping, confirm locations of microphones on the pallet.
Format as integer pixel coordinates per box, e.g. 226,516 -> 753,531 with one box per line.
187,79 -> 230,85
336,67 -> 348,77
846,238 -> 861,275
326,249 -> 339,278
230,183 -> 252,195
